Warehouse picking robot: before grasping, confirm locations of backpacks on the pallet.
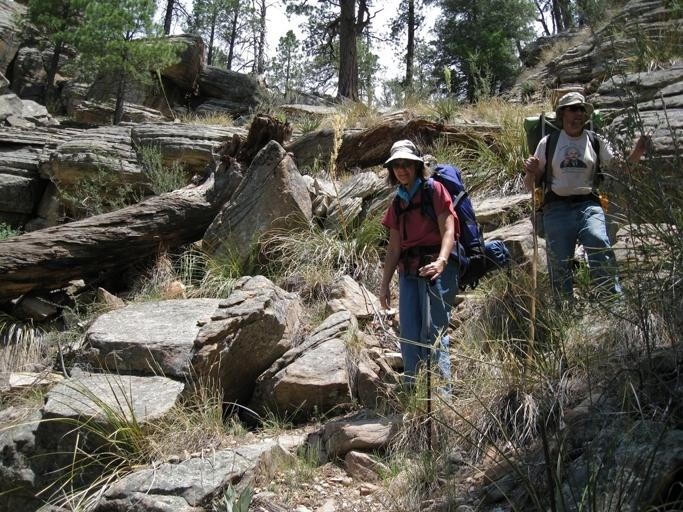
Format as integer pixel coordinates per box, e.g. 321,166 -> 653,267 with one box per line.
392,164 -> 486,291
524,114 -> 601,239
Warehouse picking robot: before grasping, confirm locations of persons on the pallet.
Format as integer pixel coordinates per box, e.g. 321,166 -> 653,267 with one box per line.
377,138 -> 460,403
517,91 -> 653,314
559,147 -> 587,169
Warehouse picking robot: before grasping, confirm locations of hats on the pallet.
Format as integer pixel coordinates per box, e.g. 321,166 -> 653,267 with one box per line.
556,92 -> 594,122
382,139 -> 424,168
